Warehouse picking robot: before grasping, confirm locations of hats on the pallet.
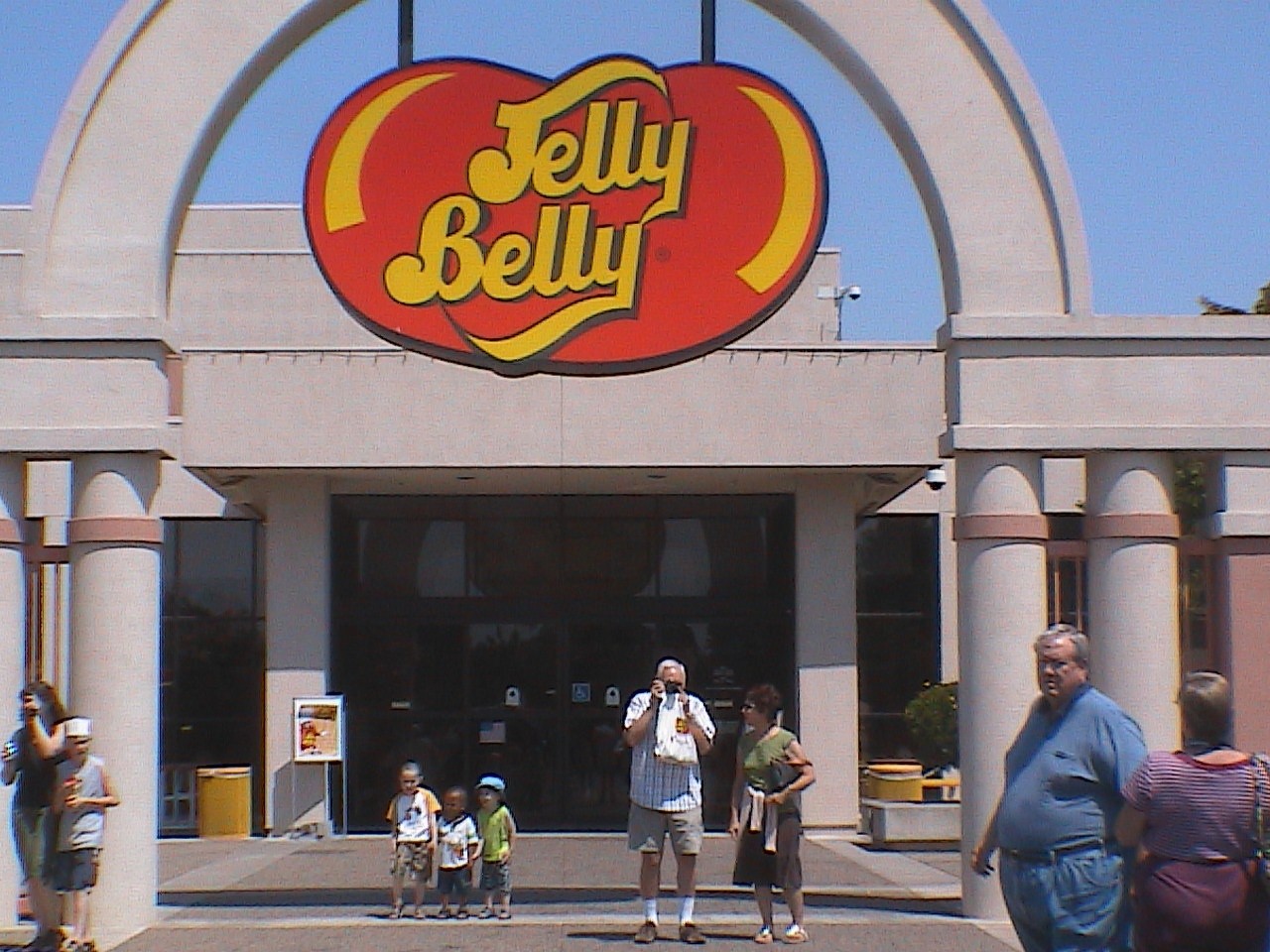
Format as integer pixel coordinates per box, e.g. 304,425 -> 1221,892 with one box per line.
474,776 -> 506,793
64,718 -> 92,737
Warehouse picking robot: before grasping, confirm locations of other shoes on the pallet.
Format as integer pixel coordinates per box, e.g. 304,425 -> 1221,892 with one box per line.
60,939 -> 98,952
678,922 -> 707,944
18,928 -> 68,952
754,924 -> 774,943
634,920 -> 657,944
414,905 -> 425,918
498,908 -> 511,919
437,908 -> 451,918
479,906 -> 497,918
455,909 -> 468,919
388,904 -> 404,919
781,923 -> 808,943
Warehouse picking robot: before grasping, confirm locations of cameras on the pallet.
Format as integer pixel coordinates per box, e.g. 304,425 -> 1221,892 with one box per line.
665,681 -> 682,694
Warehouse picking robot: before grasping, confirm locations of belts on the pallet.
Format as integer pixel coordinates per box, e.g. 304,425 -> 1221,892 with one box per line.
998,837 -> 1114,863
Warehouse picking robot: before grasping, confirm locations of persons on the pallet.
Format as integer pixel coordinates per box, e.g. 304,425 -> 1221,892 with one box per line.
620,659 -> 716,944
971,623 -> 1148,952
435,786 -> 479,920
1110,670 -> 1270,952
50,715 -> 121,952
476,777 -> 517,921
385,762 -> 442,920
728,685 -> 816,945
0,682 -> 71,952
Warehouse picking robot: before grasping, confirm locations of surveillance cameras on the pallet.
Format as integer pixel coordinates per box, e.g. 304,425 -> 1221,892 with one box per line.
925,469 -> 947,490
849,286 -> 860,299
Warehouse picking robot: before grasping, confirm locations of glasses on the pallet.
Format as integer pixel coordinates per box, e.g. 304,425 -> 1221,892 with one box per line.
741,704 -> 755,709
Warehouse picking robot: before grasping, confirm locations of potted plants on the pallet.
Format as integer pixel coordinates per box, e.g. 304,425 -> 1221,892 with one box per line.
899,681 -> 959,801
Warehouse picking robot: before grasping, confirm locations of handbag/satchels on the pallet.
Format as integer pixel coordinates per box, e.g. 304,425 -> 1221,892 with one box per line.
652,691 -> 699,767
770,756 -> 813,791
1251,750 -> 1270,894
